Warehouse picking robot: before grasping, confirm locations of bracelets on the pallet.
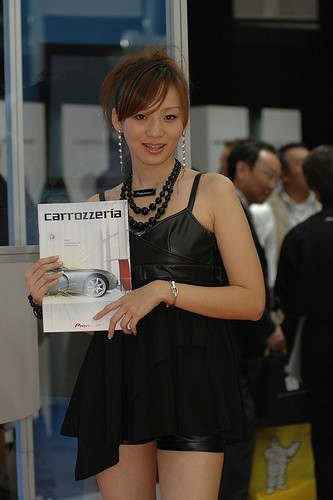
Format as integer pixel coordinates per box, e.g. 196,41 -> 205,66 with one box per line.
166,280 -> 180,308
27,294 -> 43,311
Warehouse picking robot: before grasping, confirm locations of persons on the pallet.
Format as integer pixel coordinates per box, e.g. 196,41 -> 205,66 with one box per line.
273,147 -> 333,499
22,43 -> 266,500
219,137 -> 323,499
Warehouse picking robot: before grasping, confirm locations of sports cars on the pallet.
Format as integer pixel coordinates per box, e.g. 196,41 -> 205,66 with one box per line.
48,266 -> 118,299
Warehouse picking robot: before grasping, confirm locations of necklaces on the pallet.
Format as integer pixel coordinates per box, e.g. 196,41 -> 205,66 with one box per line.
118,157 -> 181,230
